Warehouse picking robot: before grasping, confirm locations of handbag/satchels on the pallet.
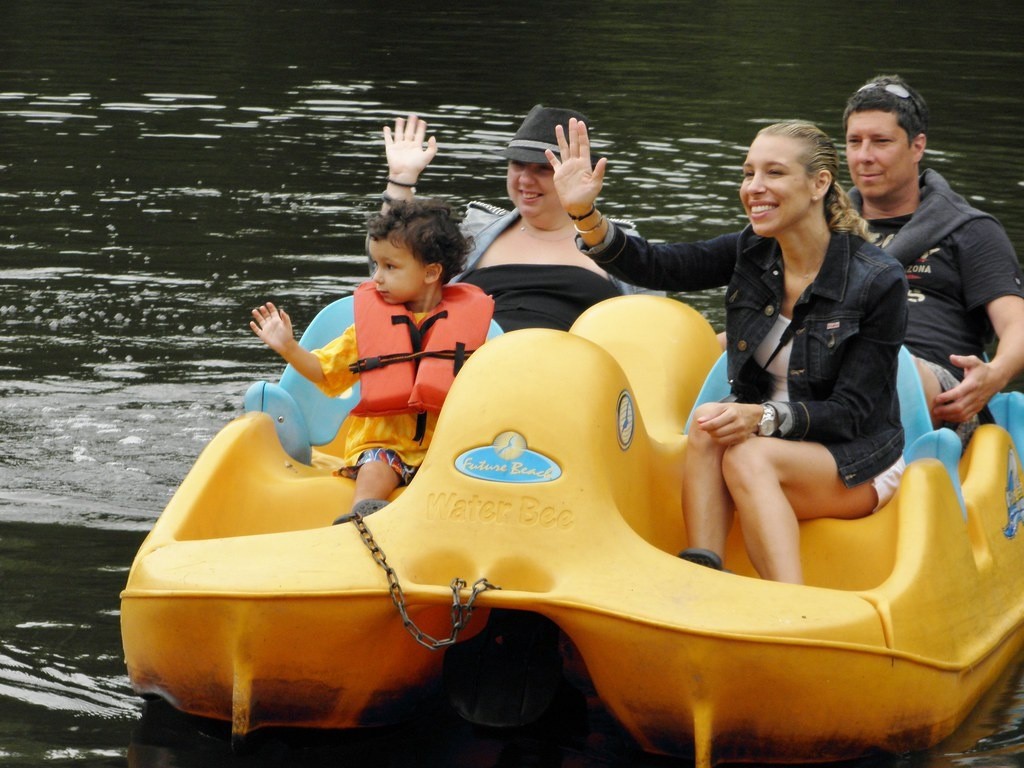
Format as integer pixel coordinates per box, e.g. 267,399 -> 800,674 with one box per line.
734,319 -> 793,404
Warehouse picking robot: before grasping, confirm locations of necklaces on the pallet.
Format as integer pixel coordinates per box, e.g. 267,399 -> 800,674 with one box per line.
783,251 -> 825,278
520,219 -> 576,242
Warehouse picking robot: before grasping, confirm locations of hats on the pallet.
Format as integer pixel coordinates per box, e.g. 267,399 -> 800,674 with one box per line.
492,104 -> 590,164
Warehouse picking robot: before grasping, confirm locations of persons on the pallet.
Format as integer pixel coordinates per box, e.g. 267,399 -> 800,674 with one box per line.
250,198 -> 495,525
545,117 -> 908,584
844,76 -> 1024,459
383,103 -> 647,333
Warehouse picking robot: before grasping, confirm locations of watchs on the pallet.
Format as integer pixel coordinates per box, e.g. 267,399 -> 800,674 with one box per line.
758,404 -> 776,436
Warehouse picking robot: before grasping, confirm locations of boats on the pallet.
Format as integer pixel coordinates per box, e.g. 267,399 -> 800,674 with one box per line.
119,293 -> 1023,768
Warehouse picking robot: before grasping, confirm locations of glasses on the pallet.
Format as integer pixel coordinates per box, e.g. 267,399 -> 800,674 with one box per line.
856,83 -> 921,121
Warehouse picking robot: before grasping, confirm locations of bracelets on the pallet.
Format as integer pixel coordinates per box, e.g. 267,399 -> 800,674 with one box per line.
568,202 -> 595,221
385,175 -> 419,186
573,209 -> 603,234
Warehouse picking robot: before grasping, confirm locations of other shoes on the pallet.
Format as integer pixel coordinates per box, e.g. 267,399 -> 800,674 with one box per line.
677,548 -> 722,570
332,499 -> 390,526
